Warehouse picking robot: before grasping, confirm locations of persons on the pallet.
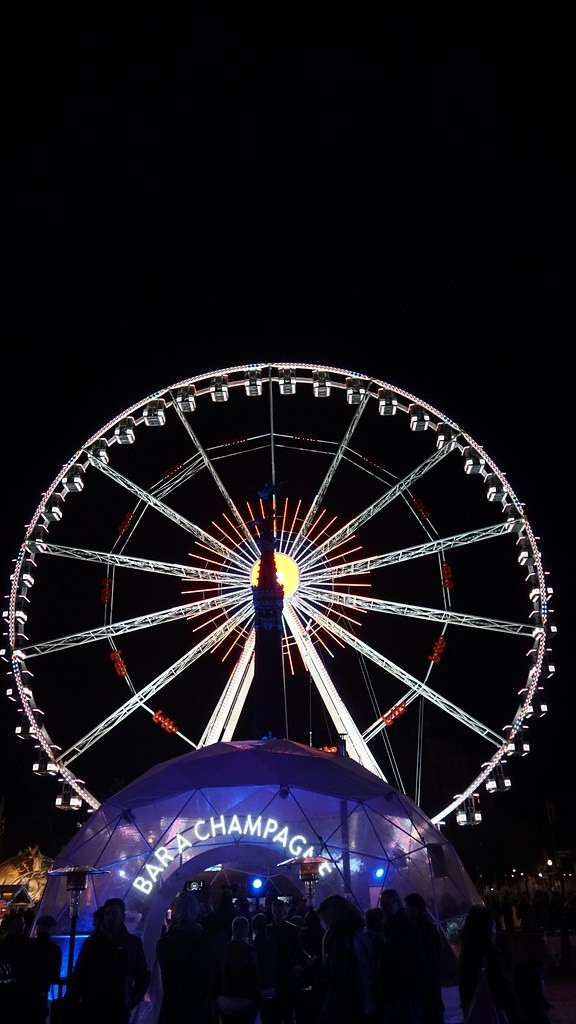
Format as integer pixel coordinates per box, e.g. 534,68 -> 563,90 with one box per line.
0,884 -> 576,1024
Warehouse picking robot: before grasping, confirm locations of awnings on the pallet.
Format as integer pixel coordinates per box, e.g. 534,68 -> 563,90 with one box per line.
30,738 -> 498,958
